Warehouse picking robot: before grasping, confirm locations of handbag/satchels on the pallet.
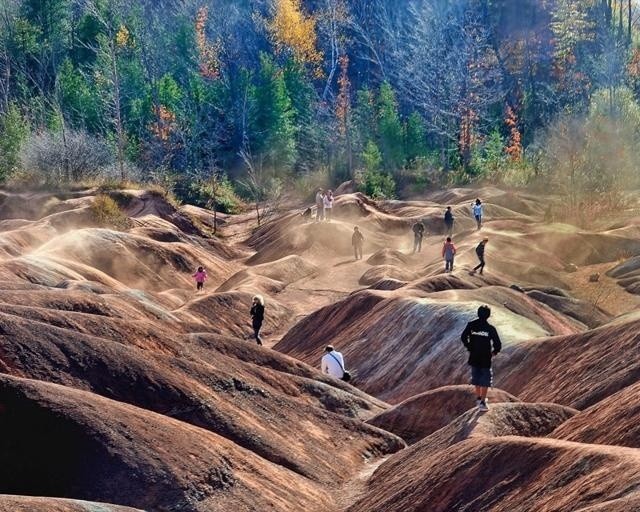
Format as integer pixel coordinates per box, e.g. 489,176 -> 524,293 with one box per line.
341,369 -> 351,382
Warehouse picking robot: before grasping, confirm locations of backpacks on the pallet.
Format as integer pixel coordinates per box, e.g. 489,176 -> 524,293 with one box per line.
445,245 -> 453,262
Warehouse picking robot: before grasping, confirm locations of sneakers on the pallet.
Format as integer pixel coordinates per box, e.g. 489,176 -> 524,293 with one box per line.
474,398 -> 490,412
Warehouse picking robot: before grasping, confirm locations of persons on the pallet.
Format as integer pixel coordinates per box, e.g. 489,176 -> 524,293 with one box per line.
472,237 -> 488,275
461,304 -> 502,411
444,206 -> 455,238
441,237 -> 457,273
321,344 -> 351,382
323,189 -> 334,222
315,188 -> 326,222
249,295 -> 265,345
191,265 -> 208,291
351,226 -> 365,260
471,199 -> 482,230
412,219 -> 424,253
